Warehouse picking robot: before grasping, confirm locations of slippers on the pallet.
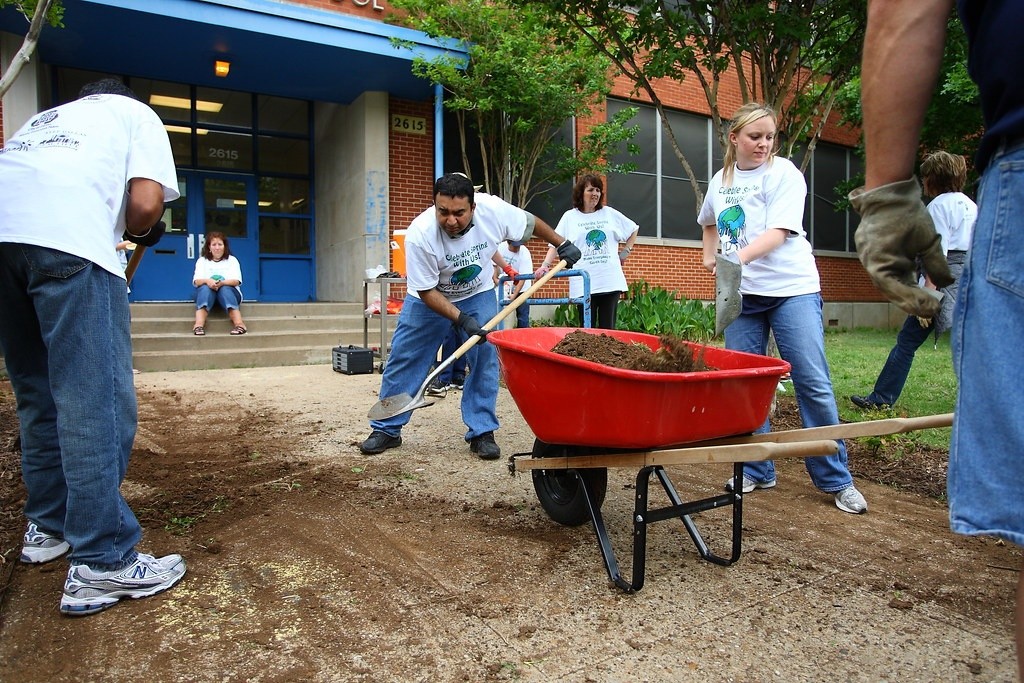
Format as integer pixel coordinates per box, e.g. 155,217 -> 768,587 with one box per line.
230,326 -> 247,335
193,326 -> 206,335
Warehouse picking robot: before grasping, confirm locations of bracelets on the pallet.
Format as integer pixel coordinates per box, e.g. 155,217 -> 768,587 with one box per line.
556,240 -> 566,249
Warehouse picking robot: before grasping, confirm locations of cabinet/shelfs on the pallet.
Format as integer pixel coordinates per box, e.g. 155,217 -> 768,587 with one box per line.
364,277 -> 409,373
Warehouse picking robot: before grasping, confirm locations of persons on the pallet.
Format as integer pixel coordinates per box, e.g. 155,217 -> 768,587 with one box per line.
360,173 -> 582,460
0,77 -> 186,614
192,231 -> 247,335
426,172 -> 483,393
696,103 -> 868,514
847,0 -> 1024,548
535,173 -> 640,330
492,239 -> 533,328
850,150 -> 978,412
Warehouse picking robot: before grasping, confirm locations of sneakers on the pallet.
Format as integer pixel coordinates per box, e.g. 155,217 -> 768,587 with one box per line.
470,431 -> 500,460
824,485 -> 867,514
446,376 -> 464,389
851,394 -> 883,411
724,474 -> 778,493
360,431 -> 402,454
426,381 -> 451,393
60,553 -> 187,616
21,519 -> 72,562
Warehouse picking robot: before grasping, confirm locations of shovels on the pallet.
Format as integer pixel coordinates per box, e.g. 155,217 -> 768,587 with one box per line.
366,259 -> 568,420
714,251 -> 742,339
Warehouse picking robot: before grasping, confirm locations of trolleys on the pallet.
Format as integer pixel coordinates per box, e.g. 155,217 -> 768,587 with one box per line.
363,275 -> 407,374
483,326 -> 955,596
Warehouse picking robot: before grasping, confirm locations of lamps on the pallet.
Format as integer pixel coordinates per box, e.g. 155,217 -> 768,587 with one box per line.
215,61 -> 230,76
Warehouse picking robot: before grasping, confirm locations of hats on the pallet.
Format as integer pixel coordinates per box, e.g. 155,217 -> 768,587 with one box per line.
507,239 -> 525,247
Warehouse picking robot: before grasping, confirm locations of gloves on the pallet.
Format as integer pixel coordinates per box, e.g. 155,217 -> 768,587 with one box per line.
619,248 -> 630,265
535,262 -> 550,280
503,265 -> 520,285
910,313 -> 931,329
848,175 -> 956,320
124,220 -> 167,247
556,238 -> 582,268
456,312 -> 493,345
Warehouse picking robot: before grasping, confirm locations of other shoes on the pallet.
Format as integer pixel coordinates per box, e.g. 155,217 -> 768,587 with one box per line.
132,369 -> 141,374
